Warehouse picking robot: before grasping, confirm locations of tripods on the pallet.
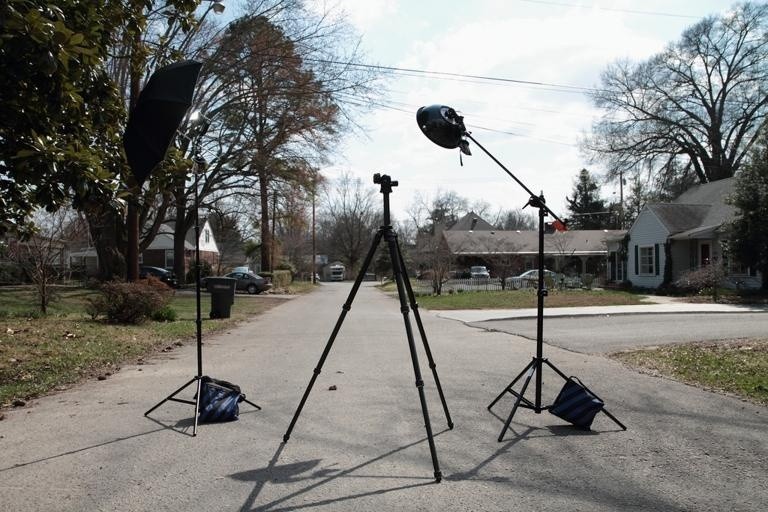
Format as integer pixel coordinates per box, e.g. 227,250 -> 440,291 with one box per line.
465,130 -> 627,442
283,173 -> 454,483
144,145 -> 262,436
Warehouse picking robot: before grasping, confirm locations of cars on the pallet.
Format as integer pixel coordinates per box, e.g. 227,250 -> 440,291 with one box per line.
199,270 -> 274,295
504,268 -> 557,290
139,265 -> 173,286
165,266 -> 180,288
307,273 -> 321,281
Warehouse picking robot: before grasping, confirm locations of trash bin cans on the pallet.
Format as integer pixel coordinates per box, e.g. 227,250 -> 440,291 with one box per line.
204,277 -> 236,318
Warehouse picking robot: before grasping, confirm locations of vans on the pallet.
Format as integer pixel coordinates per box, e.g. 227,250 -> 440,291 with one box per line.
468,265 -> 491,284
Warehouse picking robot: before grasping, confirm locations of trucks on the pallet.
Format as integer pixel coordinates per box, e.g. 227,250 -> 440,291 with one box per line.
329,263 -> 345,282
